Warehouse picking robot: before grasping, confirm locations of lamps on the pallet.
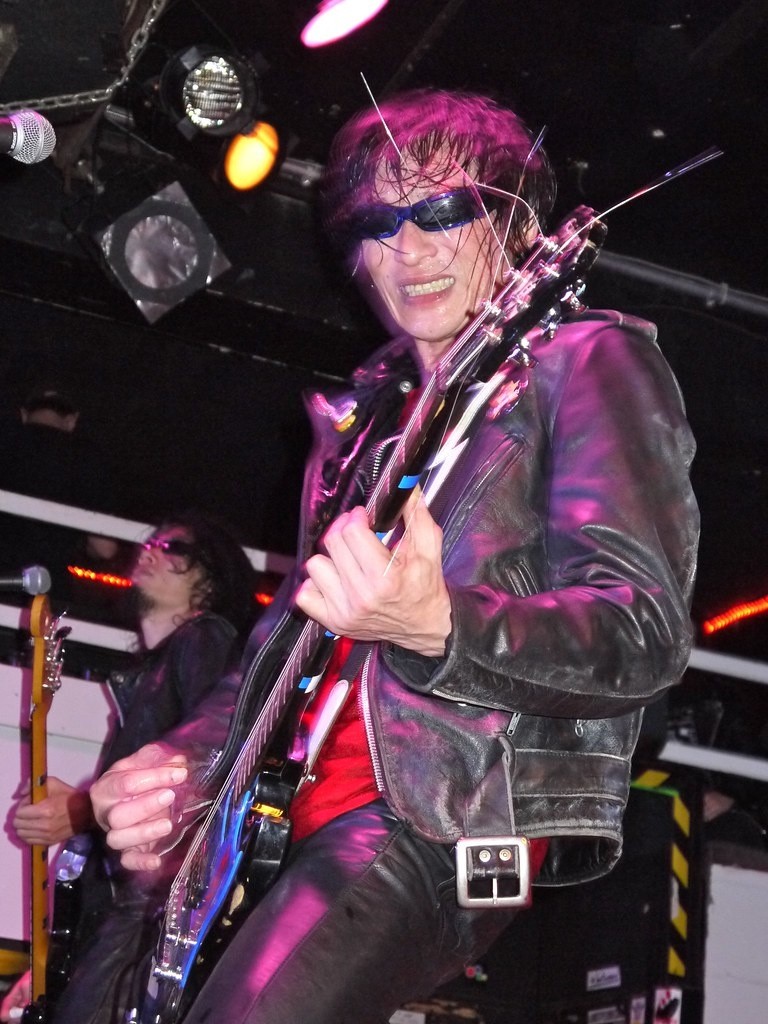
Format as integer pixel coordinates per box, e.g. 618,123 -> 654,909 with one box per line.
158,46 -> 266,142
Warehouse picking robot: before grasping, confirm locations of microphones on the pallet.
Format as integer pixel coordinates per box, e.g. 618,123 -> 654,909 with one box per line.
0,109 -> 57,163
0,565 -> 54,595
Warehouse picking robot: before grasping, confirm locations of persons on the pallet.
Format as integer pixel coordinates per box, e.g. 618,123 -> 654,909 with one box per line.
13,510 -> 264,1024
0,375 -> 116,679
91,88 -> 700,1021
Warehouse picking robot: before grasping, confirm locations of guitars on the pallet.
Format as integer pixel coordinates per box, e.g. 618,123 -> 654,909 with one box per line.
134,209 -> 611,1024
21,599 -> 68,1024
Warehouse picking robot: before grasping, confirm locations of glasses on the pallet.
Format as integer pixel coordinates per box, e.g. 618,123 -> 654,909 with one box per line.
353,180 -> 508,237
143,537 -> 208,568
